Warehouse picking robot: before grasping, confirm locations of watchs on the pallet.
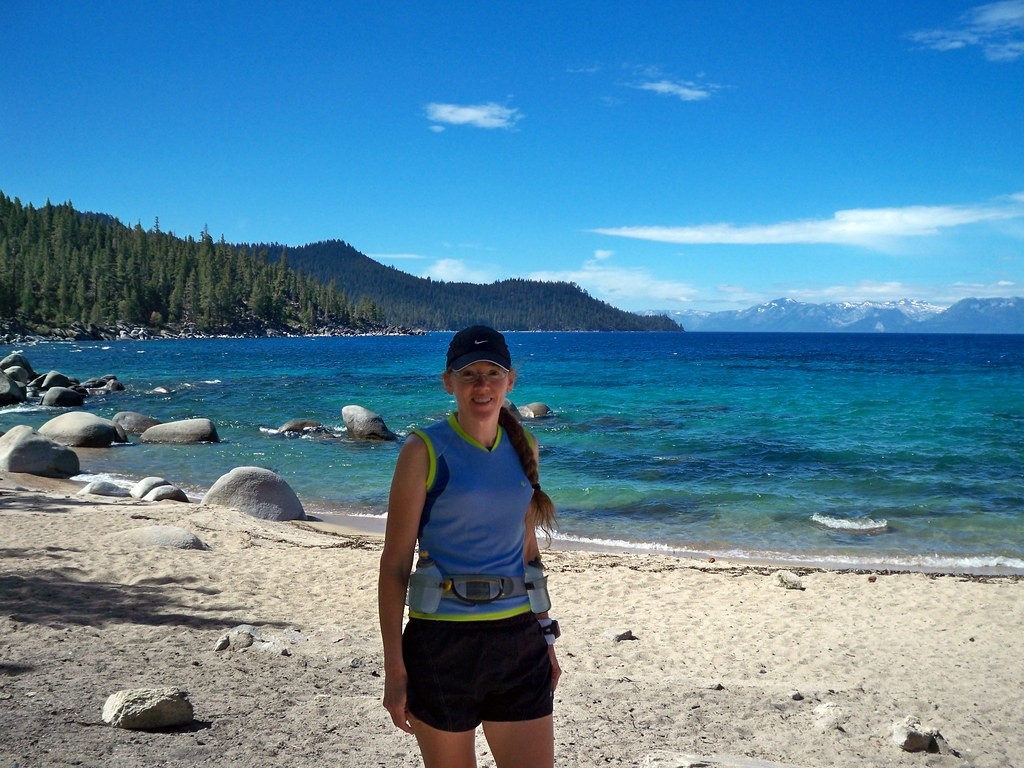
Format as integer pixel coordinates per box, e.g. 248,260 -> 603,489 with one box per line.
542,620 -> 561,639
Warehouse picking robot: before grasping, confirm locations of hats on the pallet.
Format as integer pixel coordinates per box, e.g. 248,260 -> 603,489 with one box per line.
446,325 -> 511,371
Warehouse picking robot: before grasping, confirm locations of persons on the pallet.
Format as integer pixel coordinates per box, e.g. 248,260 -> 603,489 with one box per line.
378,327 -> 562,768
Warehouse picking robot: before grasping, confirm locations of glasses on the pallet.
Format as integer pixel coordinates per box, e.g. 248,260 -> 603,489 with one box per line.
454,370 -> 508,383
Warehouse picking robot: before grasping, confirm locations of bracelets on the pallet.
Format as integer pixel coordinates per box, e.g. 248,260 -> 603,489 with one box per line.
537,618 -> 556,645
